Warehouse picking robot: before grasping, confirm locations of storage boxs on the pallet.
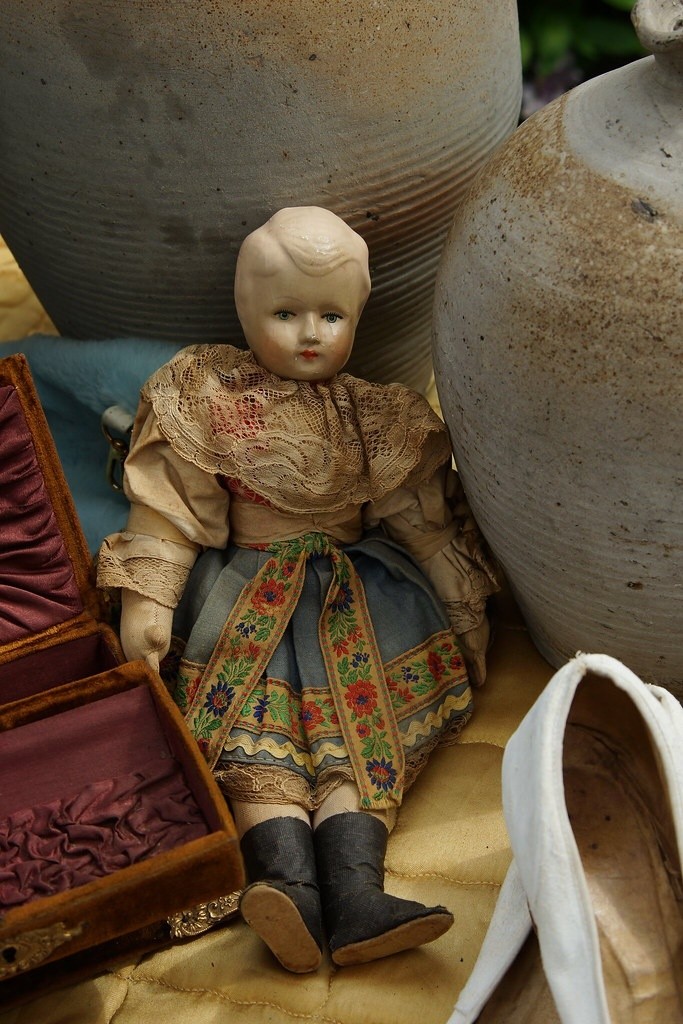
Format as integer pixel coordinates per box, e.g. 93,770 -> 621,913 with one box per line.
0,352 -> 249,982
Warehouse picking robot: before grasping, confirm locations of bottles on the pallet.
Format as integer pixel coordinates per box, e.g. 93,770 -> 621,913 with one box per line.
425,1 -> 683,692
0,0 -> 519,370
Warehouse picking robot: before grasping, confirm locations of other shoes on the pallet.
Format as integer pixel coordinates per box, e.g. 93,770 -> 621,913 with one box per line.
503,651 -> 683,1024
446,683 -> 683,1024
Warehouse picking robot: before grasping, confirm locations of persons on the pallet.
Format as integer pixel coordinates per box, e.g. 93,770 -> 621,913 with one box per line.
96,203 -> 495,972
